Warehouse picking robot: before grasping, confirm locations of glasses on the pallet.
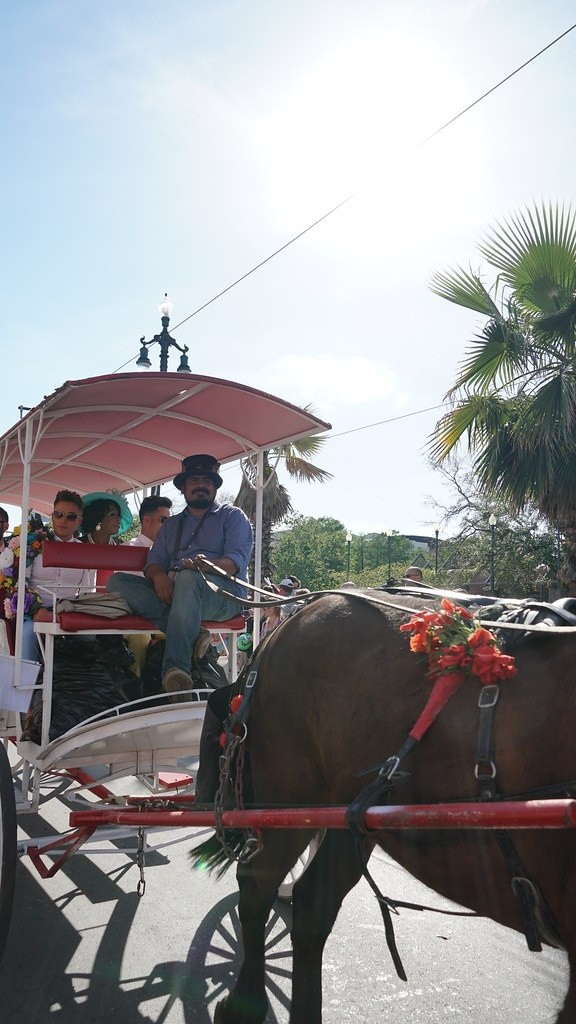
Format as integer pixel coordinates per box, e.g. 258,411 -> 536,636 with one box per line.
403,573 -> 418,579
159,516 -> 167,523
52,510 -> 79,521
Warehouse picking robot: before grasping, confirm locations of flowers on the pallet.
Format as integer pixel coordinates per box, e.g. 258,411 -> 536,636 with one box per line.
0,511 -> 50,621
394,596 -> 517,694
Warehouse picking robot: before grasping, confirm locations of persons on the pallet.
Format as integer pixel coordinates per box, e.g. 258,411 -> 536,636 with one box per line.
238,575 -> 311,658
106,454 -> 254,703
0,507 -> 10,555
14,490 -> 98,662
76,488 -> 134,545
404,567 -> 423,586
114,495 -> 172,678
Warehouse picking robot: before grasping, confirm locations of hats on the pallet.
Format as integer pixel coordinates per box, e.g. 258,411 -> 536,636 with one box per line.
77,487 -> 133,535
289,575 -> 302,589
173,454 -> 223,489
278,579 -> 294,588
236,633 -> 252,650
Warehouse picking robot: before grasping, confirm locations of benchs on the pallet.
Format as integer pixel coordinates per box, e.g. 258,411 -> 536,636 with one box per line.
28,540 -> 260,639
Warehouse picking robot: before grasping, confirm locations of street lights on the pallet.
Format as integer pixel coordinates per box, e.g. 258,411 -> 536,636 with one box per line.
387,530 -> 392,579
486,511 -> 497,591
137,291 -> 192,496
345,530 -> 352,581
434,529 -> 439,574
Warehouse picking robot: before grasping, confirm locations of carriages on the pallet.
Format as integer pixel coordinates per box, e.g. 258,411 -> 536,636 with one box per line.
0,367 -> 576,1023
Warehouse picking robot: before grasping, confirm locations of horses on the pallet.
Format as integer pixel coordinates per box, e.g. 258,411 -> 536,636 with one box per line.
193,587 -> 576,1024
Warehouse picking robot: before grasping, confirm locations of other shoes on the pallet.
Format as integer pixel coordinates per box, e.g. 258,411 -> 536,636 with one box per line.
193,630 -> 212,659
162,668 -> 193,703
20,717 -> 40,742
